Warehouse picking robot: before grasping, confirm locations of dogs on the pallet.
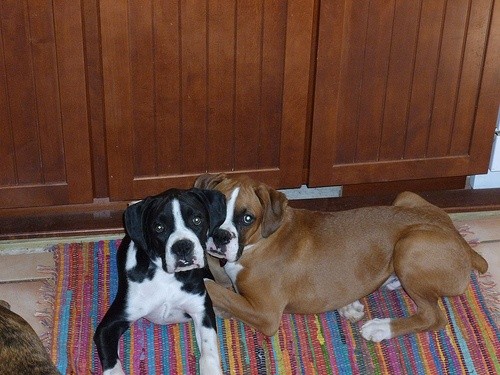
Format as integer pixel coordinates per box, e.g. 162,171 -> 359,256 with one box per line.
91,188 -> 227,375
193,172 -> 488,344
0,300 -> 64,375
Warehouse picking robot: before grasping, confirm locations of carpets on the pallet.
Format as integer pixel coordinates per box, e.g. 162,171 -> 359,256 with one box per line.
35,223 -> 500,375
